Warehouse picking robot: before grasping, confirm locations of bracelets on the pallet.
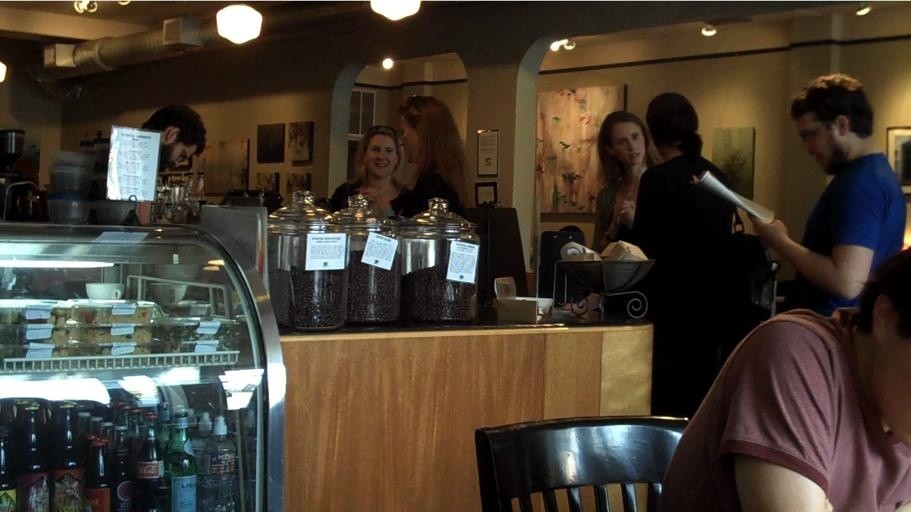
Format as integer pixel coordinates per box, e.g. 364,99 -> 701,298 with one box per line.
603,230 -> 614,243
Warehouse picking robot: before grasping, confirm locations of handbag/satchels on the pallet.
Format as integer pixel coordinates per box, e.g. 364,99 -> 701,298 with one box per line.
731,232 -> 781,334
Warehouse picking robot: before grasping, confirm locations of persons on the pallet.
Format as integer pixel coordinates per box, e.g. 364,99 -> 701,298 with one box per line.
750,74 -> 907,317
591,110 -> 661,251
327,126 -> 411,219
392,94 -> 465,216
660,247 -> 911,512
135,105 -> 207,226
563,93 -> 737,419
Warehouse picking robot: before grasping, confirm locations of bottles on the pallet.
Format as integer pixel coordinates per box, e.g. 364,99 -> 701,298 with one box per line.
0,398 -> 238,512
266,191 -> 482,330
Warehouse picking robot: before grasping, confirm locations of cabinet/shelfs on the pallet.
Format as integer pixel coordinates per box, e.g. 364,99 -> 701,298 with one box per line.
0,222 -> 289,512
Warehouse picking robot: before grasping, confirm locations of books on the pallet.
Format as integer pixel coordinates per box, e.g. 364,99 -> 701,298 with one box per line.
688,168 -> 776,223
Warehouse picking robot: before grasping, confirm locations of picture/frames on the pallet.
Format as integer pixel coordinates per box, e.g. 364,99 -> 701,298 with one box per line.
886,125 -> 911,198
475,181 -> 499,206
477,129 -> 500,177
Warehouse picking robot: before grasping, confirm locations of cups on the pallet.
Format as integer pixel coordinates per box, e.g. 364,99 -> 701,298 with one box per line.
150,283 -> 187,302
85,281 -> 124,299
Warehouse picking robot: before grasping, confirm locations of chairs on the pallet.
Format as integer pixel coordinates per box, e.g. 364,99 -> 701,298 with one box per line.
473,409 -> 692,512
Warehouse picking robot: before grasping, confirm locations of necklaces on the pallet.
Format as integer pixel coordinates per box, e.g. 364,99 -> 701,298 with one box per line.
618,178 -> 635,201
